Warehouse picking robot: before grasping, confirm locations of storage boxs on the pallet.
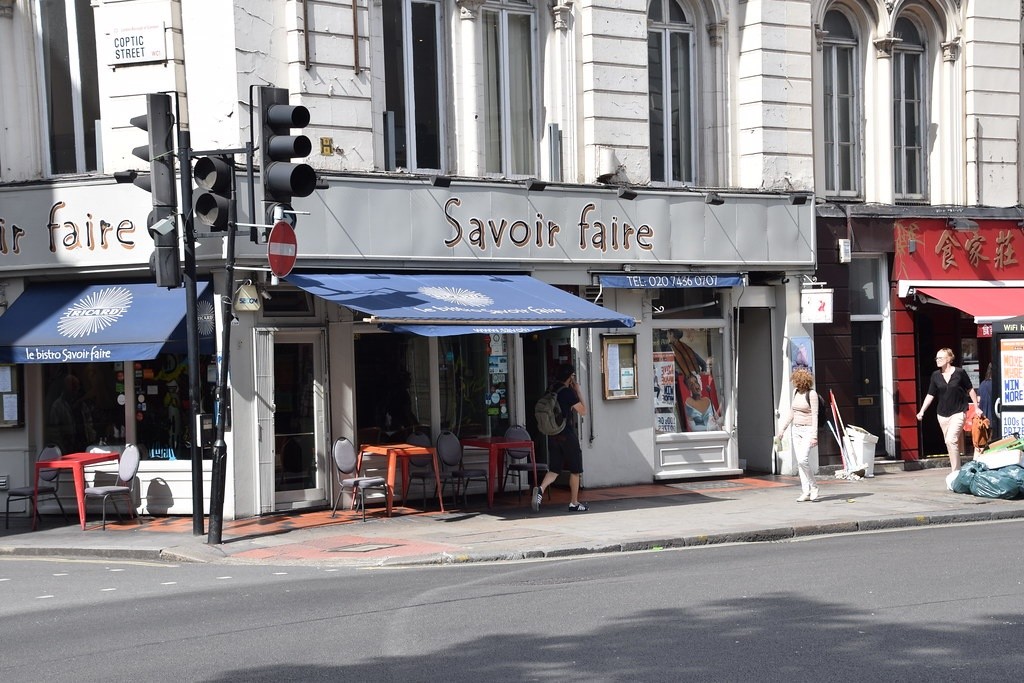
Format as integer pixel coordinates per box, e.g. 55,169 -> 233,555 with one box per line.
980,436 -> 1024,470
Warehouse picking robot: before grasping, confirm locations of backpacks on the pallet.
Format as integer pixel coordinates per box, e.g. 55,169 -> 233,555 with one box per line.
535,384 -> 568,436
795,389 -> 826,427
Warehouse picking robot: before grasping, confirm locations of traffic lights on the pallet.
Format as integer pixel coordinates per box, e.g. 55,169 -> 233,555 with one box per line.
130,91 -> 184,291
192,153 -> 237,235
257,84 -> 318,244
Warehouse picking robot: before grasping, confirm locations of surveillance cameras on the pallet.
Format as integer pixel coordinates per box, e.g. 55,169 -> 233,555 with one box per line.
149,219 -> 175,237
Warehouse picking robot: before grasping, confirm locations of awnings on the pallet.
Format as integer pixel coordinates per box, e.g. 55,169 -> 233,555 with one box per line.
0,281 -> 214,366
282,273 -> 637,338
912,287 -> 1024,339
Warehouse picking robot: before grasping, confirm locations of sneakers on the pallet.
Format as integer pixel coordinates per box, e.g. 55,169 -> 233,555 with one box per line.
569,503 -> 590,511
531,486 -> 543,513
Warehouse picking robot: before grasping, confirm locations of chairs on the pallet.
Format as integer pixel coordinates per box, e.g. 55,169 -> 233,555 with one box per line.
501,424 -> 551,503
7,443 -> 67,530
81,445 -> 143,531
437,432 -> 490,509
404,433 -> 459,510
332,436 -> 388,522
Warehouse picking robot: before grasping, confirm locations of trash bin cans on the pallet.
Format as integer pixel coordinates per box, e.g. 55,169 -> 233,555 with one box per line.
843,425 -> 879,477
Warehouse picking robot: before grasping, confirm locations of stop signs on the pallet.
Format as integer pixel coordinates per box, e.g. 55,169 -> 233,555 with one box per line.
267,222 -> 299,278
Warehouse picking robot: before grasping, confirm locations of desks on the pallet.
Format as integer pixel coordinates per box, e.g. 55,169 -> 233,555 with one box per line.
31,452 -> 133,530
352,445 -> 444,512
460,436 -> 537,509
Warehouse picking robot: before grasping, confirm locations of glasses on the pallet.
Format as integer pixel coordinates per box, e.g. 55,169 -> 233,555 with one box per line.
935,357 -> 950,362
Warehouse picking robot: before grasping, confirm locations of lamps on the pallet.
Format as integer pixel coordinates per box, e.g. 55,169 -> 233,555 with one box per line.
511,178 -> 546,192
947,217 -> 978,232
416,175 -> 452,187
114,170 -> 151,183
315,175 -> 330,189
772,190 -> 807,205
600,183 -> 637,200
686,187 -> 725,205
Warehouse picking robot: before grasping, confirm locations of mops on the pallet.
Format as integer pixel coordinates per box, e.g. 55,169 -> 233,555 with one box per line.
826,420 -> 867,481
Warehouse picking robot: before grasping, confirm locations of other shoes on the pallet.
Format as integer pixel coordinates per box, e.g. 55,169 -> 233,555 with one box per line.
810,486 -> 820,500
796,494 -> 811,502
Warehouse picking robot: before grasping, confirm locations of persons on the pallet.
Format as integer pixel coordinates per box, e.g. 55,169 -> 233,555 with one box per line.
531,362 -> 589,512
977,363 -> 998,443
776,370 -> 820,502
916,347 -> 983,472
